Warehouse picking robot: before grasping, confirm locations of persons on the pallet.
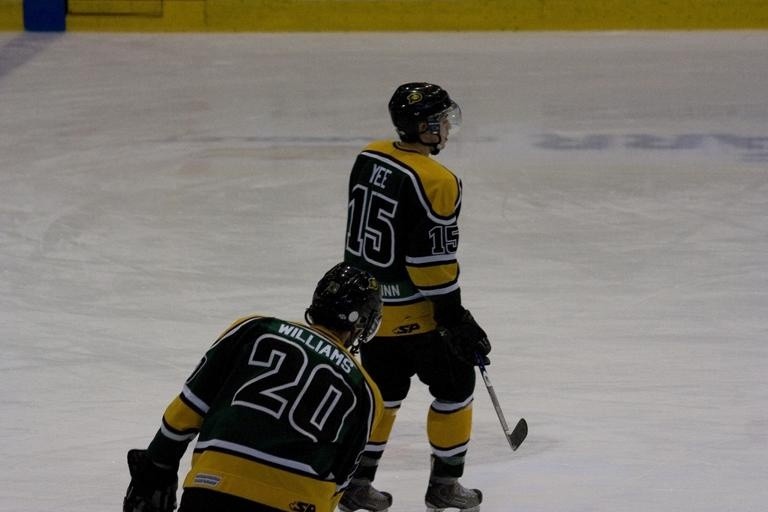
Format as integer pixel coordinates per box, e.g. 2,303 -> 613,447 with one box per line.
121,256 -> 386,511
339,75 -> 493,512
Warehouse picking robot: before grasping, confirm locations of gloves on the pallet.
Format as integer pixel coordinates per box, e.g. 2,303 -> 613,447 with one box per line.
434,306 -> 494,367
121,445 -> 179,511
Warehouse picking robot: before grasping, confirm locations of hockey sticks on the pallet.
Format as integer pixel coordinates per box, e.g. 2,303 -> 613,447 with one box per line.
476,352 -> 528,451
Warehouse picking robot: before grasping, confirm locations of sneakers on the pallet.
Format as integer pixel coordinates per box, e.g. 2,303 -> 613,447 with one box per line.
423,476 -> 483,510
338,483 -> 393,512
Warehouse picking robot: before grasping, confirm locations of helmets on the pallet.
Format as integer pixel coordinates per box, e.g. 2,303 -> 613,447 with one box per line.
308,257 -> 386,350
386,81 -> 454,146
